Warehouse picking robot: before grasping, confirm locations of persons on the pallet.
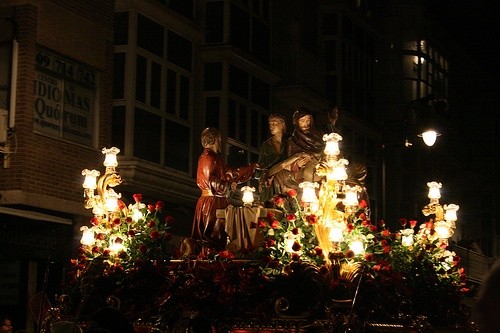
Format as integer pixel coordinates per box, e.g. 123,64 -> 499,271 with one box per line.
190,99 -> 369,259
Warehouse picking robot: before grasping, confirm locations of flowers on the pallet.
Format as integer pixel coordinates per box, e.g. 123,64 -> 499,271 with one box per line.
70,193 -> 174,281
248,187 -> 472,316
